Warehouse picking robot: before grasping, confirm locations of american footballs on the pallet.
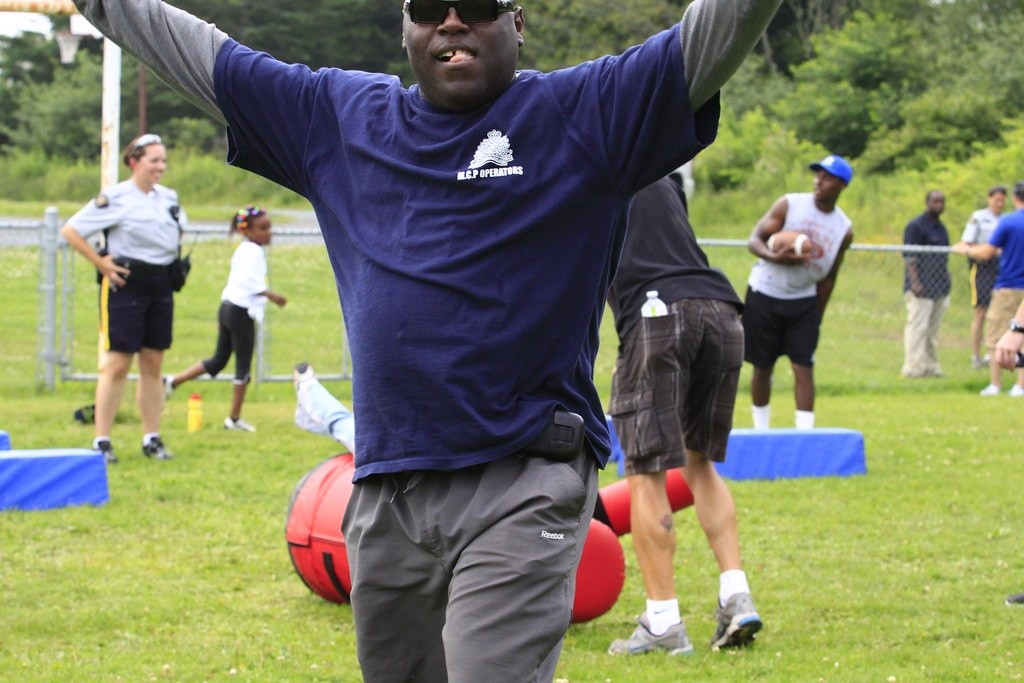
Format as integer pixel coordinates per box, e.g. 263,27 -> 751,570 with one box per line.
765,231 -> 812,254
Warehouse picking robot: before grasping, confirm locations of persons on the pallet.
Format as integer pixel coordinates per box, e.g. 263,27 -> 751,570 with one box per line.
995,300 -> 1024,603
954,184 -> 1024,396
962,187 -> 1008,369
608,177 -> 764,656
902,191 -> 951,377
164,207 -> 286,432
62,134 -> 188,462
293,361 -> 355,454
74,0 -> 784,683
741,157 -> 853,430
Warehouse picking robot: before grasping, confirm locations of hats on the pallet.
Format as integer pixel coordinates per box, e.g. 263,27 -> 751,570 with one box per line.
809,155 -> 853,185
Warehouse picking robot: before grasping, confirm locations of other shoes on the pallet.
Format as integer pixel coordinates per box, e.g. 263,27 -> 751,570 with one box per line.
981,384 -> 999,395
225,416 -> 255,433
1009,383 -> 1024,396
162,375 -> 175,401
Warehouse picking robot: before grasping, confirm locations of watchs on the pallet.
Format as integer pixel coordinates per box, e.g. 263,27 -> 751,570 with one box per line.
1008,320 -> 1024,333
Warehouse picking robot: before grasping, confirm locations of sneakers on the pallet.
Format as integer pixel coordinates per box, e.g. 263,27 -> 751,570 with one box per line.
608,612 -> 691,656
142,436 -> 172,460
95,440 -> 117,463
710,591 -> 762,650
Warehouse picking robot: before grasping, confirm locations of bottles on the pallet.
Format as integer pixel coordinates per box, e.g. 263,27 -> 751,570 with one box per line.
642,290 -> 668,318
188,393 -> 202,431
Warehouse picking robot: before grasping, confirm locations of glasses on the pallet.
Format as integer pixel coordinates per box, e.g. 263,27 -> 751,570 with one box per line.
130,134 -> 161,156
405,0 -> 517,24
